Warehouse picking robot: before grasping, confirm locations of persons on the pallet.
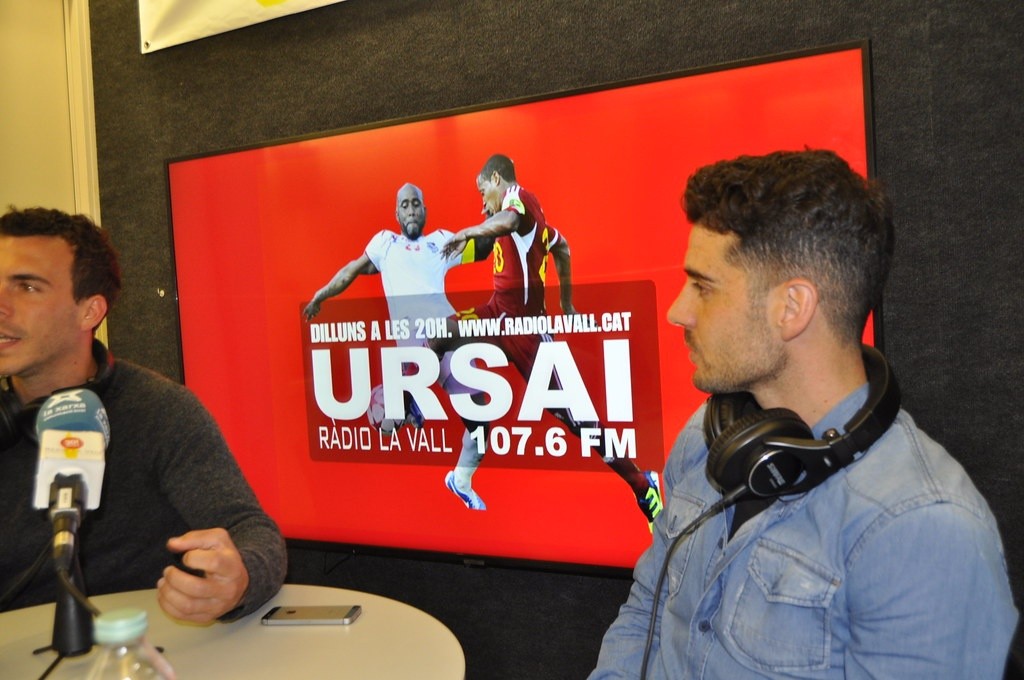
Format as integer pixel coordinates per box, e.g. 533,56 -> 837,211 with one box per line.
0,208 -> 288,627
587,134 -> 1024,680
303,155 -> 670,540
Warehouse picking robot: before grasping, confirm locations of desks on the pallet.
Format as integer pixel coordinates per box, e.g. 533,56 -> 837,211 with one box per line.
0,585 -> 466,680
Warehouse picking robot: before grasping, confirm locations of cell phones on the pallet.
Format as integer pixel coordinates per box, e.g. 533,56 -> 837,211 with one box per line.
262,605 -> 361,626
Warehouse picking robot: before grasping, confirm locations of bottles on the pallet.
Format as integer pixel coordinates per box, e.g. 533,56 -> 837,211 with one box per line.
83,607 -> 180,680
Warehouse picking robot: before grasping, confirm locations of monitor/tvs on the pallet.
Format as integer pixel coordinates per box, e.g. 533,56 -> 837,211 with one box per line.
166,35 -> 886,580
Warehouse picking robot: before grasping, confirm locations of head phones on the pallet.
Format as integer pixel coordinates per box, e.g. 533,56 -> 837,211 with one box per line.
0,339 -> 116,447
702,343 -> 901,501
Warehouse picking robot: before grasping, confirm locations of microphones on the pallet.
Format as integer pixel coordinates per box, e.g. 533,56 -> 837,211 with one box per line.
33,388 -> 111,573
